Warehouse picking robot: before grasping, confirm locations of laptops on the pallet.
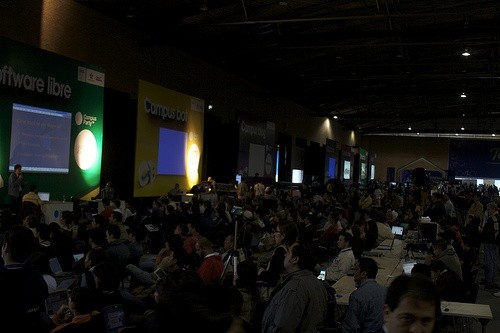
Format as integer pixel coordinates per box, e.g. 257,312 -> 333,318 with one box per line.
391,225 -> 404,236
317,267 -> 327,280
72,252 -> 85,263
402,260 -> 423,274
44,289 -> 71,319
37,191 -> 50,203
144,223 -> 159,232
377,238 -> 395,250
154,267 -> 167,279
102,303 -> 127,331
48,256 -> 66,274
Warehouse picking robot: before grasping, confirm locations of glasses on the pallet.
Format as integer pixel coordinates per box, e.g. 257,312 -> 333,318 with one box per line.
273,229 -> 281,233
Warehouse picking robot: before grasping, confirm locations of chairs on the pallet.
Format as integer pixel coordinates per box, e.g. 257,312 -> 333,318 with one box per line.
23,201 -> 40,219
469,283 -> 480,303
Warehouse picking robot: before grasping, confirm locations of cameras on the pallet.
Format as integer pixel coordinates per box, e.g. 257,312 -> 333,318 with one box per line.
232,206 -> 242,217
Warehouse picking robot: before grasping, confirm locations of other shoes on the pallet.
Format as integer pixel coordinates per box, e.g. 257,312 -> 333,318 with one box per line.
493,292 -> 500,297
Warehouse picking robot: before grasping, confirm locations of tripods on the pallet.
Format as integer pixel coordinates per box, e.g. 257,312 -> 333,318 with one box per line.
219,216 -> 242,288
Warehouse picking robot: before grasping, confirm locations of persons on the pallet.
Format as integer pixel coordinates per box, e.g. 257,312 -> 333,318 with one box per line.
0,164 -> 500,333
261,242 -> 327,333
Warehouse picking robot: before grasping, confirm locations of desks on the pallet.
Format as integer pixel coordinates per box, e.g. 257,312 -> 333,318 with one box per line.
440,301 -> 492,333
217,189 -> 238,205
332,238 -> 410,333
172,194 -> 217,203
40,201 -> 73,226
88,199 -> 126,215
